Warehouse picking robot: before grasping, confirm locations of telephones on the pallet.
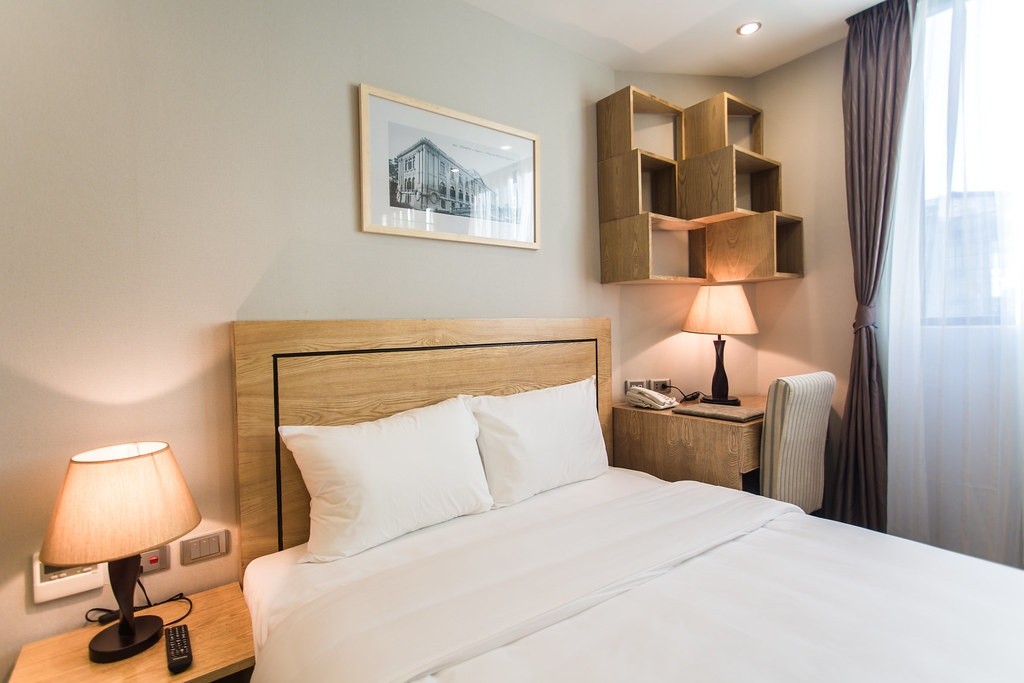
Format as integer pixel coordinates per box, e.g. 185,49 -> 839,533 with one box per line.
626,386 -> 679,411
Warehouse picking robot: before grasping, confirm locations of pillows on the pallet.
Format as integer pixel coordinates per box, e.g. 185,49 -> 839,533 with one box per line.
277,375 -> 609,564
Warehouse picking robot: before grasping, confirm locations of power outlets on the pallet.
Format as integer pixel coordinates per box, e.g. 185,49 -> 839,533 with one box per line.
650,378 -> 672,395
626,380 -> 646,391
139,545 -> 171,574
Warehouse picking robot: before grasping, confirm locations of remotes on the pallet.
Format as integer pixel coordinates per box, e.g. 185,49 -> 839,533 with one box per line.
165,624 -> 192,674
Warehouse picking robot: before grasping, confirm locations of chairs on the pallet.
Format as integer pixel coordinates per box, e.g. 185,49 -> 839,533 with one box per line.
760,371 -> 836,514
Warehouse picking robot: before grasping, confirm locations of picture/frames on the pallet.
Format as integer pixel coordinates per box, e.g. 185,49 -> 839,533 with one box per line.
359,83 -> 542,251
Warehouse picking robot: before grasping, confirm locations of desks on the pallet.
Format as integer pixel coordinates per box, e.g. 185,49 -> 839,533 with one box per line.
613,395 -> 767,491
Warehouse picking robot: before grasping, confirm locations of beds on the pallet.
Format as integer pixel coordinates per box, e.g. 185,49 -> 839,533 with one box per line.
231,316 -> 1024,683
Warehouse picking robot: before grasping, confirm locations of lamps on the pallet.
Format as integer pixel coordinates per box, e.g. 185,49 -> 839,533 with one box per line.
39,440 -> 202,663
681,284 -> 760,406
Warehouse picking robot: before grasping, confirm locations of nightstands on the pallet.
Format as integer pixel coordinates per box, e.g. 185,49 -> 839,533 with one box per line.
7,581 -> 255,683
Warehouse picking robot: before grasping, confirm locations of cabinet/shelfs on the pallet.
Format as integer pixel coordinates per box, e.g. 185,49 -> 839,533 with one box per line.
596,84 -> 805,286
